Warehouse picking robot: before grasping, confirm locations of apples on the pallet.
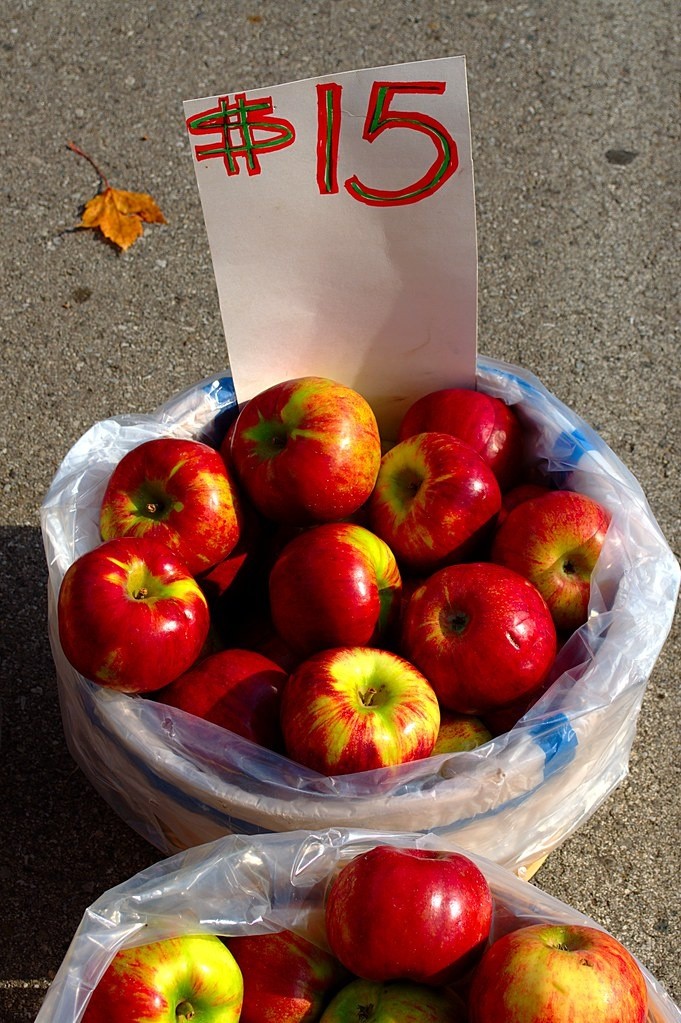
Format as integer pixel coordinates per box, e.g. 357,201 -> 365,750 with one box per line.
57,377 -> 623,782
78,844 -> 658,1023
